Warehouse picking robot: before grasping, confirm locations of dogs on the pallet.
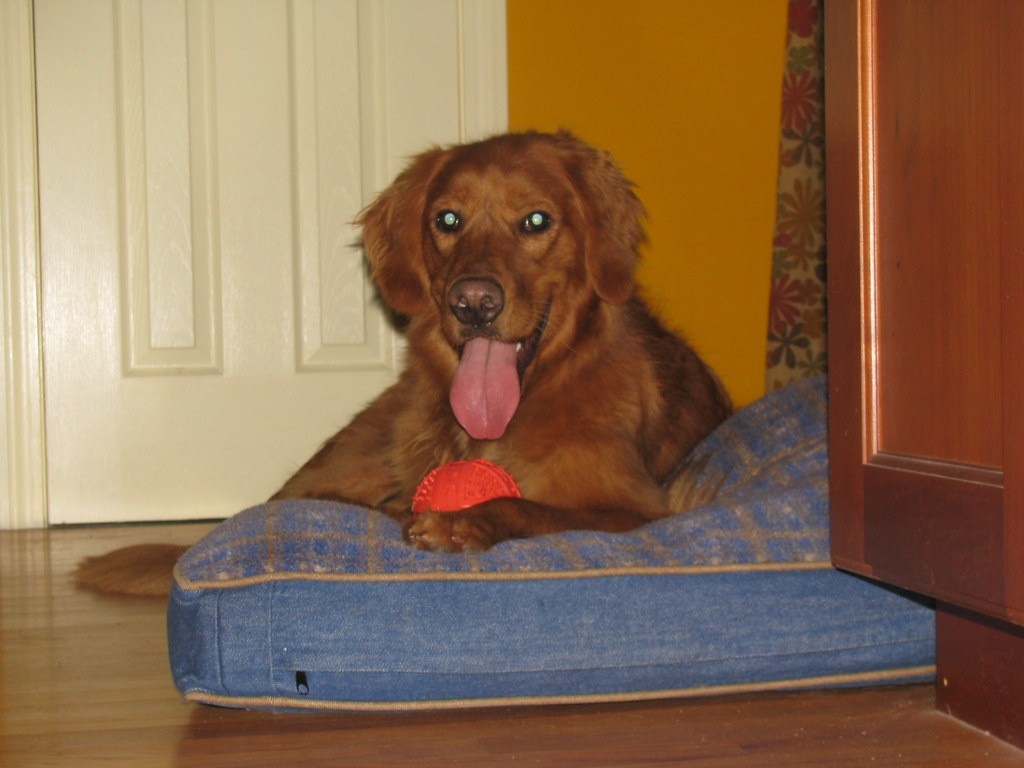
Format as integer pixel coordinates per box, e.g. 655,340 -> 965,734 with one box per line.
70,128 -> 736,597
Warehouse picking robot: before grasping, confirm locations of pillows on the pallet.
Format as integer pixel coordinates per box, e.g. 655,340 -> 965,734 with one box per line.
166,371 -> 938,715
